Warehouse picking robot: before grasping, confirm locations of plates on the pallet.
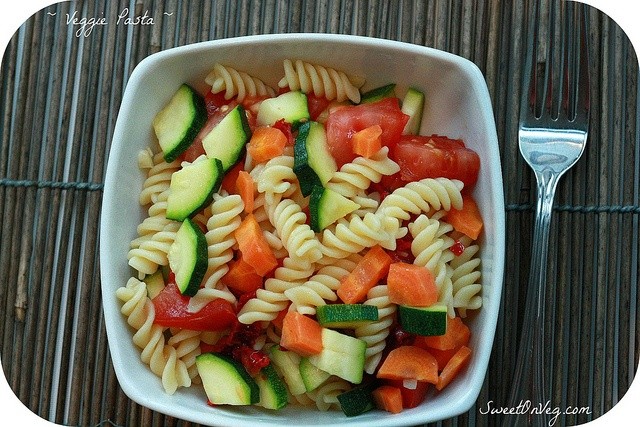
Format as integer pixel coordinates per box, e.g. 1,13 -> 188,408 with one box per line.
100,32 -> 506,426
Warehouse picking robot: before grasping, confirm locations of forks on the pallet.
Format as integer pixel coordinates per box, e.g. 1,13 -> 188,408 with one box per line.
500,0 -> 591,426
